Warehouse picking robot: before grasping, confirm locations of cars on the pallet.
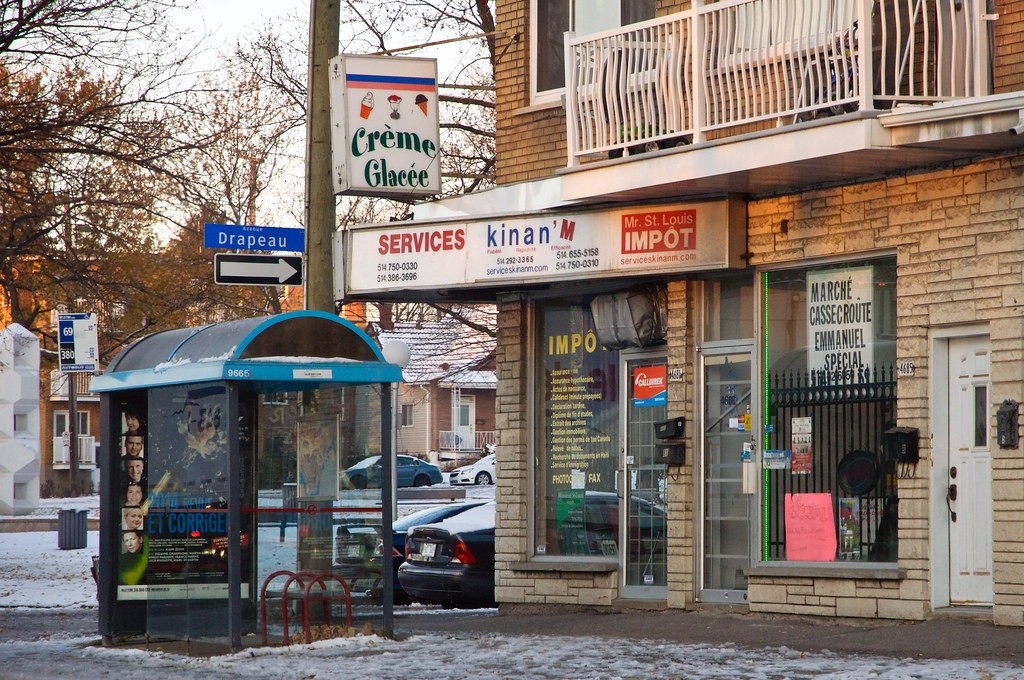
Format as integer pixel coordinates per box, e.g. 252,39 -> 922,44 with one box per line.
398,490 -> 667,607
450,453 -> 497,484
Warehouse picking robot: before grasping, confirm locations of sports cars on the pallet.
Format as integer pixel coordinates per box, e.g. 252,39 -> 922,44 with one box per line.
339,455 -> 441,489
336,503 -> 489,604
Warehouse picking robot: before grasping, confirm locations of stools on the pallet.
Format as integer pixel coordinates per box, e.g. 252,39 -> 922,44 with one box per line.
281,572 -> 352,647
261,570 -> 330,646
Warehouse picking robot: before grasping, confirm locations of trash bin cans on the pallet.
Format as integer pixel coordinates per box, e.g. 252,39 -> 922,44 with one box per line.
57,508 -> 90,549
281,483 -> 298,521
